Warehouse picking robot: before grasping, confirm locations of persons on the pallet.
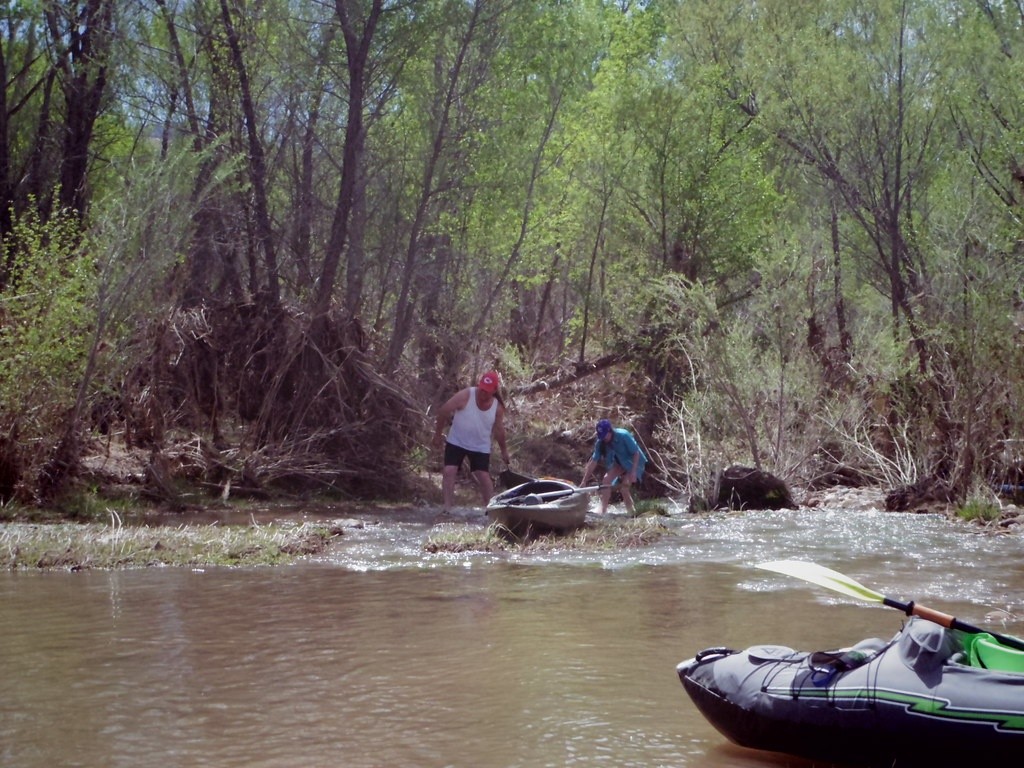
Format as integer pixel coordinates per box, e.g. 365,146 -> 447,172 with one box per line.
431,372 -> 511,518
579,419 -> 648,518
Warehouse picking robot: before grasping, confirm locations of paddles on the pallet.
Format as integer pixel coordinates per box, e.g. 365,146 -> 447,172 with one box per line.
753,555 -> 1011,645
501,485 -> 616,508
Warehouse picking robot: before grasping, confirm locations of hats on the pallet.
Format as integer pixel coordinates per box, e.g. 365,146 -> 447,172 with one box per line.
596,419 -> 611,439
479,372 -> 499,393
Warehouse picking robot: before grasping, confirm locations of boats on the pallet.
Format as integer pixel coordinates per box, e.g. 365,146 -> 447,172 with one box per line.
676,617 -> 1024,768
488,477 -> 587,542
